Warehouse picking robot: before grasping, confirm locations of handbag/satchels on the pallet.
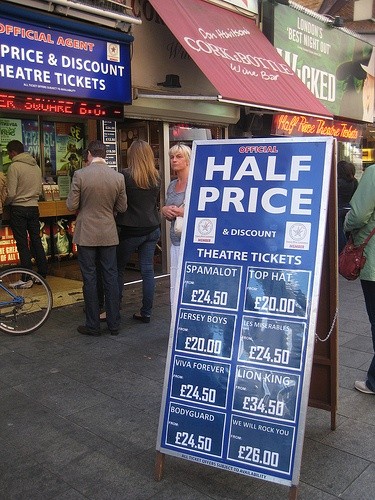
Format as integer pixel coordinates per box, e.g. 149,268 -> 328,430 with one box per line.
338,236 -> 367,280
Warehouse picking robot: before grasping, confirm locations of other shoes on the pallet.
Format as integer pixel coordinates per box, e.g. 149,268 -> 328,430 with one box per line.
132,313 -> 151,324
101,317 -> 107,321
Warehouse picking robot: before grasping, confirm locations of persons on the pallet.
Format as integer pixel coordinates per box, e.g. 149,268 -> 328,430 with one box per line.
100,140 -> 160,322
66,141 -> 127,334
338,160 -> 358,252
344,164 -> 375,394
0,140 -> 47,289
162,144 -> 191,312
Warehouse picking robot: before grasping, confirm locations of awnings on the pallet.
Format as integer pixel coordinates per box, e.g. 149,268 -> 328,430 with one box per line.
138,0 -> 333,120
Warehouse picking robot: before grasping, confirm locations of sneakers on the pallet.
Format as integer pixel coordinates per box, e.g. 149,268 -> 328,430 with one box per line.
107,326 -> 118,336
354,379 -> 375,394
78,326 -> 102,337
9,280 -> 33,289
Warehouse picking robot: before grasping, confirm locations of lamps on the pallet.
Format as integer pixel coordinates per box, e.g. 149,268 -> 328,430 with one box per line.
326,16 -> 345,27
157,74 -> 182,89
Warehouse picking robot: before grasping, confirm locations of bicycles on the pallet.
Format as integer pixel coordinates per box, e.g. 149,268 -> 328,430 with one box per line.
0,268 -> 54,336
